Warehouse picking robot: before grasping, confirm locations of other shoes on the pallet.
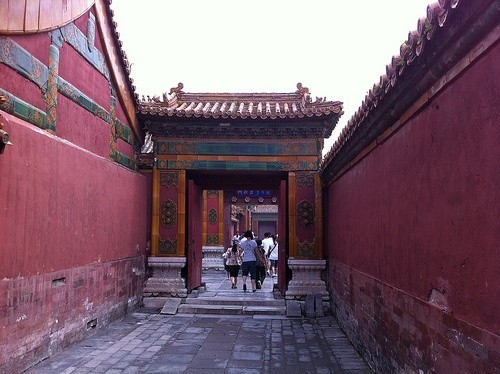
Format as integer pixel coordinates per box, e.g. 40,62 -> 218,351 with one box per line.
271,273 -> 278,278
267,269 -> 270,276
235,286 -> 237,288
231,283 -> 235,289
252,285 -> 256,292
242,283 -> 247,290
257,280 -> 262,289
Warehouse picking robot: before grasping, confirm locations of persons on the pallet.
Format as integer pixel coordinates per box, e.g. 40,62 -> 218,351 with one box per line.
238,231 -> 263,293
221,225 -> 278,289
